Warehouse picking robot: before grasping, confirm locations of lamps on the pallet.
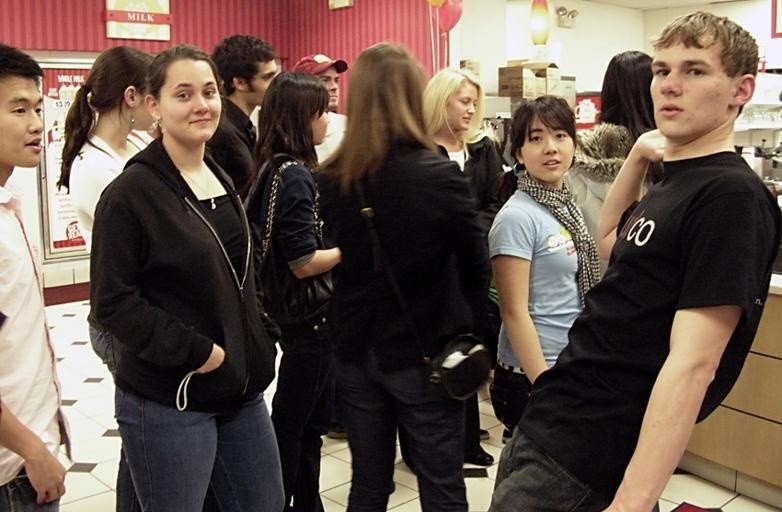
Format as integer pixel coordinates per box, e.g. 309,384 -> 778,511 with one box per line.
530,0 -> 551,46
556,7 -> 579,28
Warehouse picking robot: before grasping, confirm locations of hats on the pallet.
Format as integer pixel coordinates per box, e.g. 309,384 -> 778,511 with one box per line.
285,48 -> 350,85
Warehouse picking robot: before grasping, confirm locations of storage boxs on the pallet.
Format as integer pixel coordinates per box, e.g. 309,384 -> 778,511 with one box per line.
523,62 -> 561,98
498,66 -> 547,99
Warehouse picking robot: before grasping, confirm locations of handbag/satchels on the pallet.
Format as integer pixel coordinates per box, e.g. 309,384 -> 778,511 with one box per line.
258,161 -> 335,324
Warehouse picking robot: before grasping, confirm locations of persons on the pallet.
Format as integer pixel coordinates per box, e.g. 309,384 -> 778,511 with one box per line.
289,53 -> 346,165
313,40 -> 491,511
56,45 -> 159,511
243,70 -> 342,512
0,43 -> 72,512
422,67 -> 511,467
567,52 -> 655,271
86,45 -> 286,512
487,13 -> 780,512
205,33 -> 282,202
486,96 -> 608,442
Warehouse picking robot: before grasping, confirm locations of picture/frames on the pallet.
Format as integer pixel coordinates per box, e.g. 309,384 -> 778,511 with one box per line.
22,46 -> 102,265
327,0 -> 353,11
